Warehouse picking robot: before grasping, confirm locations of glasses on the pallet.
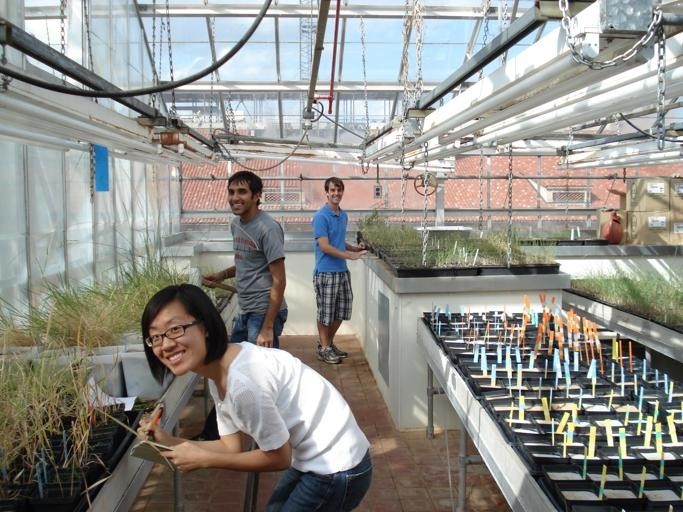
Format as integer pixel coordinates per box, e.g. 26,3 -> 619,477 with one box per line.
145,319 -> 200,348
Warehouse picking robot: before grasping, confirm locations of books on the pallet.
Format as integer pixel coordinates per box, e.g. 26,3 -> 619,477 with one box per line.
130,440 -> 176,473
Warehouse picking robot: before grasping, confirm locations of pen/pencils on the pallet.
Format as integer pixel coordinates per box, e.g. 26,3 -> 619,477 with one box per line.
147,405 -> 164,435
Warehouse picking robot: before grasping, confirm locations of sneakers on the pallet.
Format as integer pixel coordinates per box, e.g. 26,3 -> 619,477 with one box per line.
318,343 -> 347,364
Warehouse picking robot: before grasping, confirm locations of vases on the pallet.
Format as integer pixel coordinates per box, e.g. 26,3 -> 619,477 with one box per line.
423,309 -> 683,511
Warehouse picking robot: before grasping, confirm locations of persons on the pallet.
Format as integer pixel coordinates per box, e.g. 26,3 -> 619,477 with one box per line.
313,177 -> 368,364
137,284 -> 373,512
201,170 -> 288,349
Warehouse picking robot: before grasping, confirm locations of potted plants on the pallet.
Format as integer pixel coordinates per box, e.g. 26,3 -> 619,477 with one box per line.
356,208 -> 561,277
563,261 -> 683,333
0,231 -> 236,511
